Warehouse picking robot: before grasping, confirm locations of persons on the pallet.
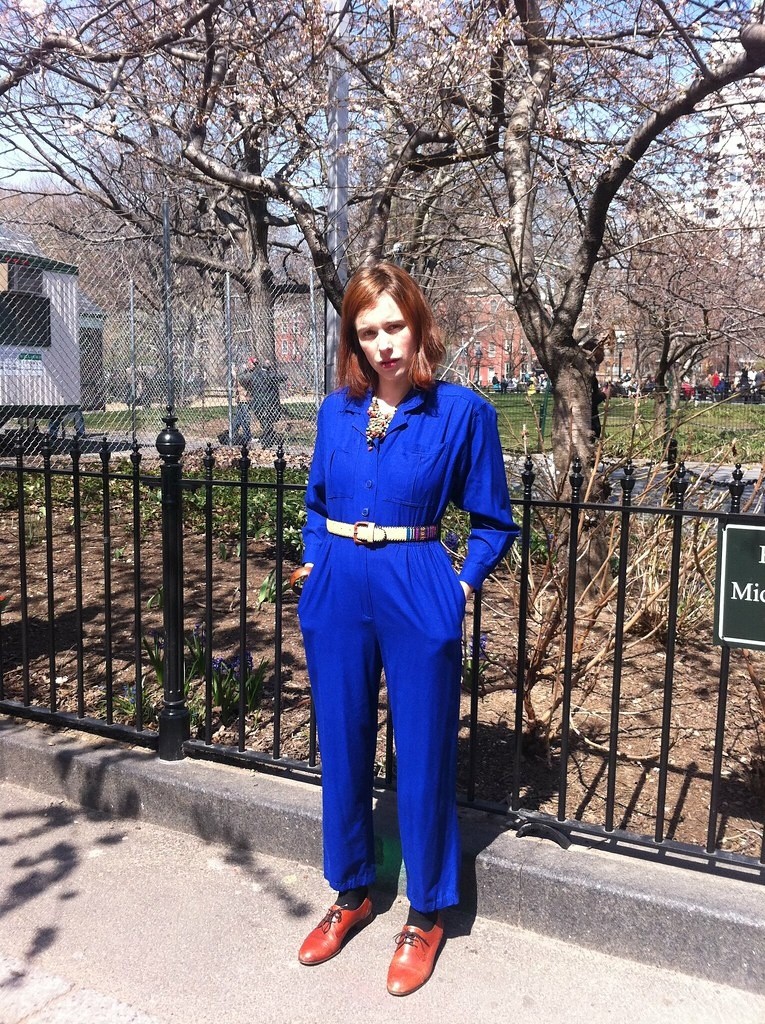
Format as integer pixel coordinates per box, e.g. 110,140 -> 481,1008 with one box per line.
619,364 -> 765,403
49,412 -> 86,437
217,359 -> 288,443
292,264 -> 518,995
493,373 -> 549,392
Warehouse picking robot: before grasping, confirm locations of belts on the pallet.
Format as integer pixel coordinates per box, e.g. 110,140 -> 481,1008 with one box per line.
326,518 -> 436,543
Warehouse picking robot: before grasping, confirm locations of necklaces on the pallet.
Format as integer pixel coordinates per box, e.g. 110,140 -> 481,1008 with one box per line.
366,394 -> 395,450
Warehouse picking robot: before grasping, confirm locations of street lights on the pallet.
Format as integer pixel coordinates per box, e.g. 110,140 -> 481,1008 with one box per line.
724,316 -> 733,382
475,349 -> 483,385
615,334 -> 626,378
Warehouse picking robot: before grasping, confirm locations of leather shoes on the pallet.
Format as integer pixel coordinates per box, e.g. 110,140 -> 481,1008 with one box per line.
299,892 -> 374,965
386,909 -> 445,996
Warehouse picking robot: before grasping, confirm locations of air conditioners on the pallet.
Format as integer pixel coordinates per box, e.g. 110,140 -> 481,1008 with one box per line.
0,263 -> 43,295
